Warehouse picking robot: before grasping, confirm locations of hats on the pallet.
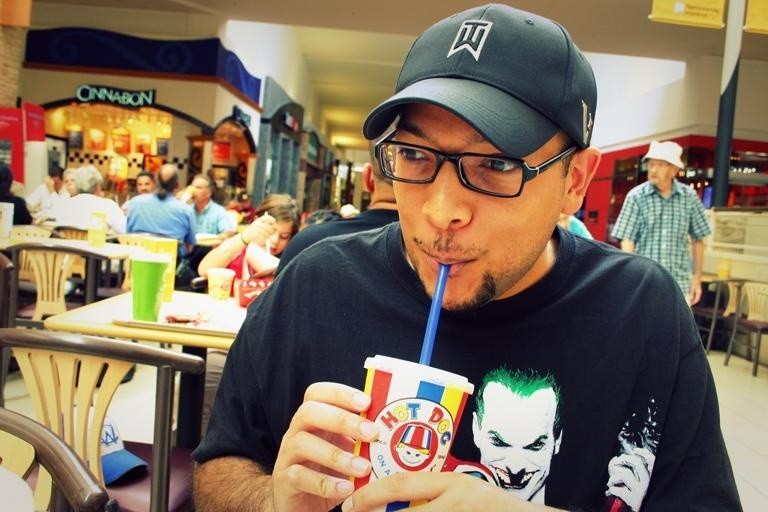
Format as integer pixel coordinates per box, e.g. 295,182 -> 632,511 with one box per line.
363,5 -> 597,158
72,406 -> 150,485
641,140 -> 685,170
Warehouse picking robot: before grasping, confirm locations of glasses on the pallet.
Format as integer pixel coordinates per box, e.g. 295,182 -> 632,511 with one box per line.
374,131 -> 578,198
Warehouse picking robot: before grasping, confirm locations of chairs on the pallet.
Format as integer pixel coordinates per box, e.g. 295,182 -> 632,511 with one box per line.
683,274 -> 767,376
0,221 -> 207,511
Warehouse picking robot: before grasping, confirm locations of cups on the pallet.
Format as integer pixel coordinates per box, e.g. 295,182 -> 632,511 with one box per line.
353,355 -> 475,511
130,255 -> 170,323
207,265 -> 236,302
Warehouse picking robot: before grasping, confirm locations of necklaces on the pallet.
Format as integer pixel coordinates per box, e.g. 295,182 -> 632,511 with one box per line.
368,198 -> 397,204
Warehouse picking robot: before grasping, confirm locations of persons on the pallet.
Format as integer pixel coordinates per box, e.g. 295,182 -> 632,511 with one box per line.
273,114 -> 400,279
192,3 -> 743,512
611,137 -> 712,310
556,209 -> 595,241
0,159 -> 360,440
470,363 -> 657,512
